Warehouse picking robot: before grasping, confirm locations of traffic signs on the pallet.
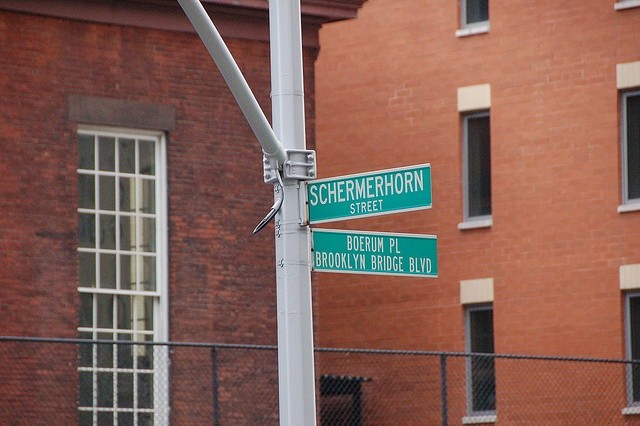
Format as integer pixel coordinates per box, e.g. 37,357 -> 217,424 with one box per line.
311,227 -> 439,280
304,163 -> 433,225
